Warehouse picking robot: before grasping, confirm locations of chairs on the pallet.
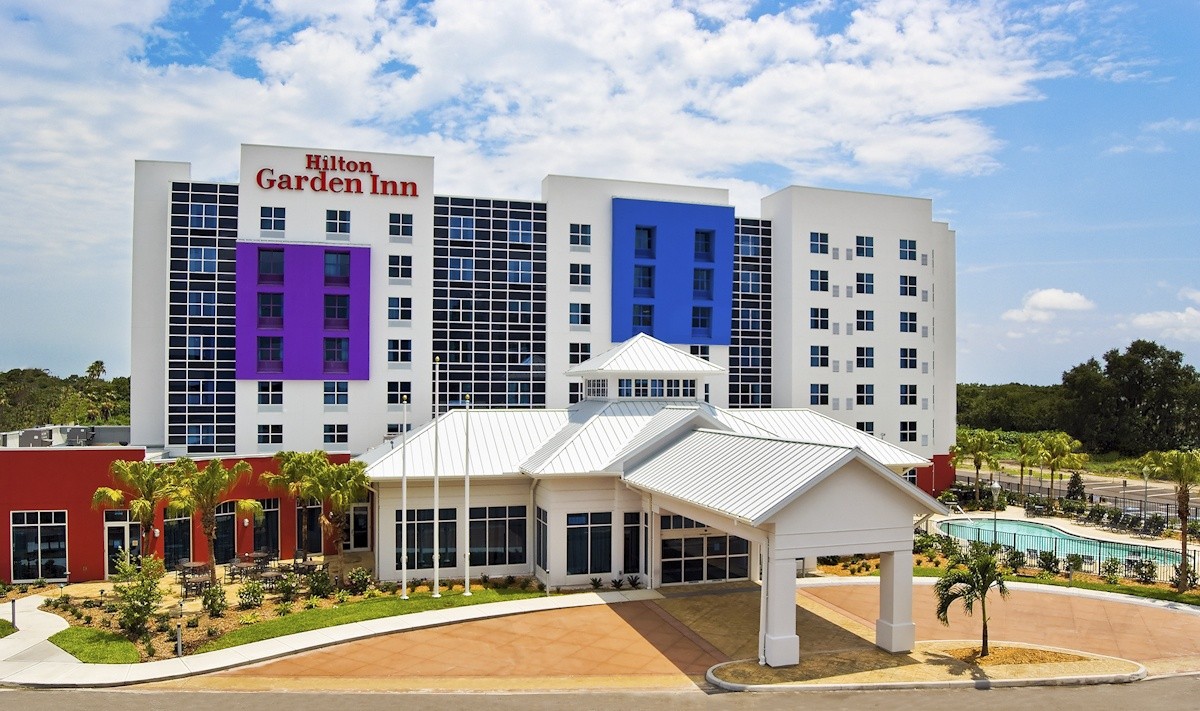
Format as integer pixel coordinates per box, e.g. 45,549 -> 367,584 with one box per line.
1003,544 -> 1142,575
172,549 -> 325,598
1026,501 -> 1162,538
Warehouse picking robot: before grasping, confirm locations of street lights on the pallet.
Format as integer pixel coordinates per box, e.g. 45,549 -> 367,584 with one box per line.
990,481 -> 1002,545
1142,465 -> 1150,521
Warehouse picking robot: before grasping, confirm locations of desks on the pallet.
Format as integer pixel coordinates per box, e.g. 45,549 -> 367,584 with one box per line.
262,572 -> 279,590
185,561 -> 206,575
303,561 -> 320,576
188,577 -> 210,594
249,552 -> 268,567
238,563 -> 257,579
